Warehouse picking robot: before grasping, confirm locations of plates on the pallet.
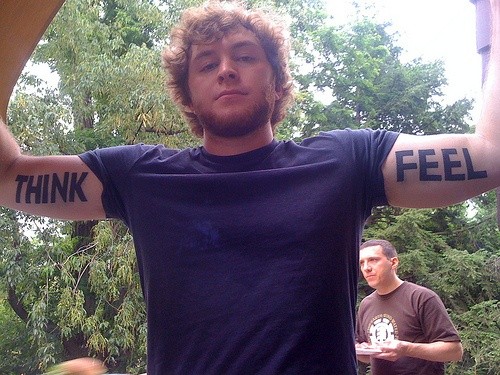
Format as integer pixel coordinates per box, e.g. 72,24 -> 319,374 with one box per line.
356,348 -> 382,355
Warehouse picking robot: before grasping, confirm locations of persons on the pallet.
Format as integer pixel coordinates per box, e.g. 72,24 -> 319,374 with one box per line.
350,238 -> 463,375
0,0 -> 500,375
